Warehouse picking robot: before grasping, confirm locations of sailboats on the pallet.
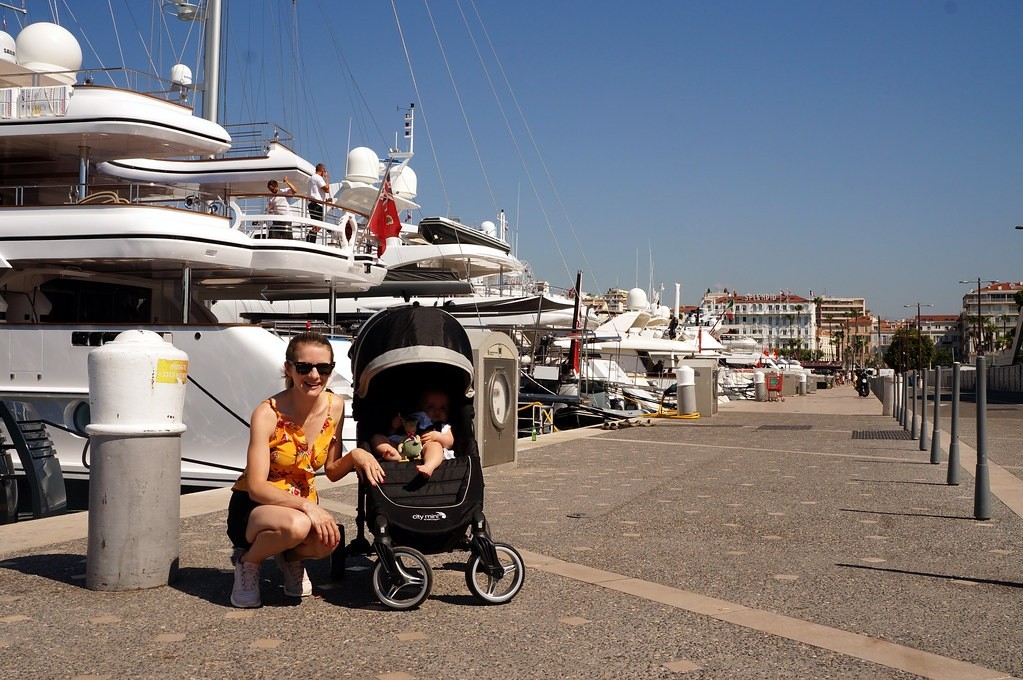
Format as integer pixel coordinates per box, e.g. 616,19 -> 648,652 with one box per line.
1,0 -> 780,523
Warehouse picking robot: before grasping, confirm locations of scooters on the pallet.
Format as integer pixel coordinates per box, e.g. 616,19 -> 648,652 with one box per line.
857,373 -> 870,397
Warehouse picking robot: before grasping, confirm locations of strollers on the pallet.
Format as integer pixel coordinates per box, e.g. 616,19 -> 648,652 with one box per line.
346,303 -> 526,610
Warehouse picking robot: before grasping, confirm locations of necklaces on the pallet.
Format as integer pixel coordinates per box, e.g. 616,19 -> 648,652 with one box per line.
308,396 -> 319,424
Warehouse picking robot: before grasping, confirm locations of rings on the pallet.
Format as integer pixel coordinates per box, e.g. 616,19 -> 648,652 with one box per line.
376,469 -> 378,470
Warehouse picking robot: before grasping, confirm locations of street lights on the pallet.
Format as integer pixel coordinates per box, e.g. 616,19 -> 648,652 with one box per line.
894,318 -> 915,374
904,302 -> 934,387
959,276 -> 1000,356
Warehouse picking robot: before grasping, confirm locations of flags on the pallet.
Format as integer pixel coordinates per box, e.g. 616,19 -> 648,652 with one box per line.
724,299 -> 733,320
366,169 -> 403,259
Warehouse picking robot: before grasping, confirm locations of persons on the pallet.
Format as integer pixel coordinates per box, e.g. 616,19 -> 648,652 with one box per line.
305,164 -> 333,243
0,266 -> 52,323
267,175 -> 297,238
371,391 -> 454,478
835,370 -> 856,387
226,332 -> 386,609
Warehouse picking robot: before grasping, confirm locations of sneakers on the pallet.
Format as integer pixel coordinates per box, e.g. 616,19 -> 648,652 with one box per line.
230,552 -> 261,607
274,552 -> 312,596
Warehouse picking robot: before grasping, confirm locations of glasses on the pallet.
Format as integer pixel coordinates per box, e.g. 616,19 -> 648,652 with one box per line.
288,360 -> 335,375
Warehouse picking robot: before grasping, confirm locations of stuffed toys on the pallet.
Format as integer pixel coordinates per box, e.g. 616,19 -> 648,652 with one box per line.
398,417 -> 423,462
